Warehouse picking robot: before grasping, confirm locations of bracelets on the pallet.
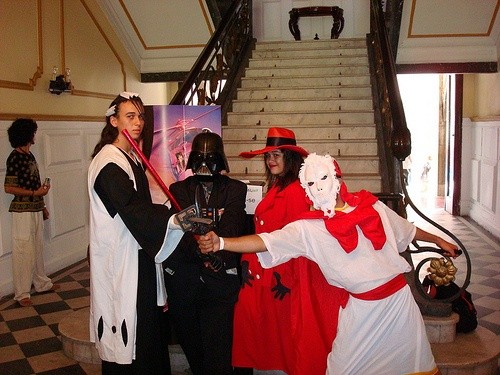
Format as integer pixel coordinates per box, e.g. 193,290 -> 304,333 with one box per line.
219,237 -> 224,250
32,189 -> 35,197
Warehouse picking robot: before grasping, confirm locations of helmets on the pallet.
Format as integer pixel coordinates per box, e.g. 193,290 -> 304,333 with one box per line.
185,133 -> 230,176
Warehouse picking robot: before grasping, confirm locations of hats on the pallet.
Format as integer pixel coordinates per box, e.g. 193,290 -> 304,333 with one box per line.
239,127 -> 309,159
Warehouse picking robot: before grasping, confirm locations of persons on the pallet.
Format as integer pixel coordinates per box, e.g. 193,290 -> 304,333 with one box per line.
198,153 -> 459,375
231,127 -> 315,375
168,131 -> 248,375
4,119 -> 61,306
87,92 -> 191,375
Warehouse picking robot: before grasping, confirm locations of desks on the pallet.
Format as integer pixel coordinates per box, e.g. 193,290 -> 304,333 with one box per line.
287,6 -> 345,40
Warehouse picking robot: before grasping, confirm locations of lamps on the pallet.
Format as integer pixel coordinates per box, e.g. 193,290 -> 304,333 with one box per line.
48,66 -> 73,96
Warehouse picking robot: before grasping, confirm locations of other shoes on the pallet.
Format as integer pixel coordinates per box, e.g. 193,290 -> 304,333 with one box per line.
50,283 -> 60,290
20,298 -> 32,307
